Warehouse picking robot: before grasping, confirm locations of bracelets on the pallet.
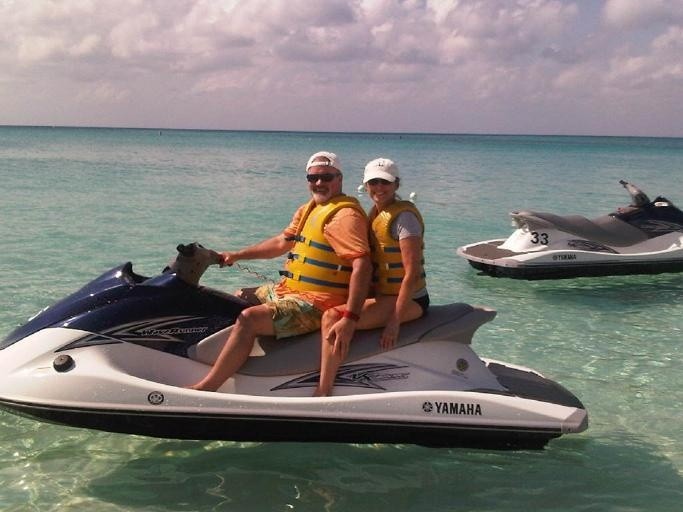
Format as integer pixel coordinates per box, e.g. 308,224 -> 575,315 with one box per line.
341,309 -> 360,322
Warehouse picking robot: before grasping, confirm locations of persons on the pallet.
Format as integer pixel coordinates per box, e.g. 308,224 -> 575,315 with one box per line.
181,149 -> 373,394
309,157 -> 430,399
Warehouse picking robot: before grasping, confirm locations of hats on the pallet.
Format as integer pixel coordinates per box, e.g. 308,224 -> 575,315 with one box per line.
305,151 -> 342,172
361,157 -> 400,184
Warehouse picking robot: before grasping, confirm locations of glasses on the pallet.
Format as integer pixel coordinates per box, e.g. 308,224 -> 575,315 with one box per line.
306,173 -> 340,183
365,178 -> 399,185
